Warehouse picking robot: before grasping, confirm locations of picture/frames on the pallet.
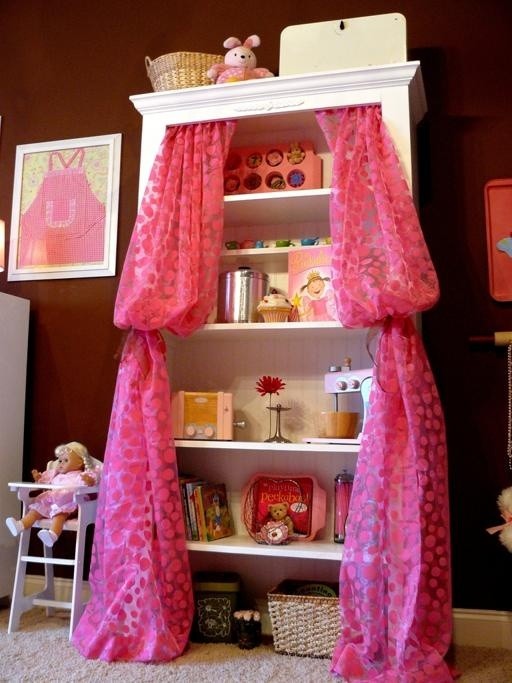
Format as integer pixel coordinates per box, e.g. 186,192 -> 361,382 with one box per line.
6,133 -> 123,282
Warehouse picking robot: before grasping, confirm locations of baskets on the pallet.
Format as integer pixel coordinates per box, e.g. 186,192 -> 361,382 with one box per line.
267,585 -> 339,658
145,51 -> 225,92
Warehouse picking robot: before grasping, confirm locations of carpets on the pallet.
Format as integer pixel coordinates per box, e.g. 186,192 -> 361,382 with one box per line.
1,625 -> 336,683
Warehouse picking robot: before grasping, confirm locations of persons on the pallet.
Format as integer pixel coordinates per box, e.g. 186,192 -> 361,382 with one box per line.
5,441 -> 98,547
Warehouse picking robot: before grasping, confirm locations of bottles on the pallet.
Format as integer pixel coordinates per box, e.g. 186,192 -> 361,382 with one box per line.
334,471 -> 355,544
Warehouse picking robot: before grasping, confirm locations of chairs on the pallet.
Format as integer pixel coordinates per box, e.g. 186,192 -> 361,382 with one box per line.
7,455 -> 104,642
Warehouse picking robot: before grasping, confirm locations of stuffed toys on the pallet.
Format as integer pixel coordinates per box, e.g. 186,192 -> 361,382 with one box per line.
207,35 -> 274,86
270,506 -> 293,537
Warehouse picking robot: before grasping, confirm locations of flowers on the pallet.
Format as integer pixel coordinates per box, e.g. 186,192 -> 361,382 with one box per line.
254,374 -> 286,440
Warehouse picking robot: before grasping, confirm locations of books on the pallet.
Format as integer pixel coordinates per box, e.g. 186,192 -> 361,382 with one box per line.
175,463 -> 234,542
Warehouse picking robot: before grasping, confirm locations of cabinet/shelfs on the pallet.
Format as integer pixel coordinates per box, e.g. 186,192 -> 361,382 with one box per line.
102,62 -> 429,562
